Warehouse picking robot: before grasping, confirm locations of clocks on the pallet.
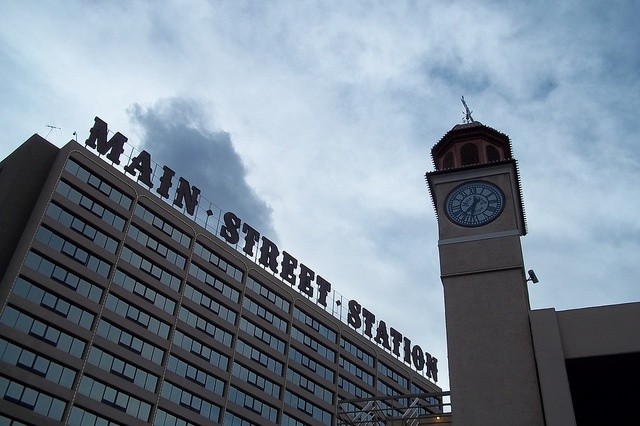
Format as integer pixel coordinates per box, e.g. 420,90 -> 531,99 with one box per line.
442,179 -> 507,228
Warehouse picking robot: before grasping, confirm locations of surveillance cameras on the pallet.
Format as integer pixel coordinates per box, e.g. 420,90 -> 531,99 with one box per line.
526,270 -> 540,283
